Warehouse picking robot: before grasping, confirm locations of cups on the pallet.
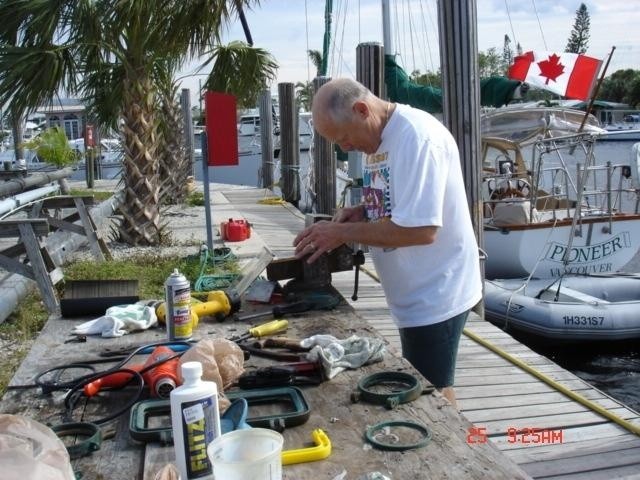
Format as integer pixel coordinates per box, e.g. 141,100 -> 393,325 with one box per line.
206,428 -> 285,479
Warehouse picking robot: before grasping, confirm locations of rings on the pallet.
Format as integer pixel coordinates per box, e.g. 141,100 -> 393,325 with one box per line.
309,243 -> 315,252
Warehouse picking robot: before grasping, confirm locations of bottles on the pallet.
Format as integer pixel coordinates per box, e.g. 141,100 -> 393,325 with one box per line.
200,242 -> 208,263
169,361 -> 221,479
165,268 -> 193,340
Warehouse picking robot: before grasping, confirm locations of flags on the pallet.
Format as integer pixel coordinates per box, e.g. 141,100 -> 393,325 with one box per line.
506,51 -> 602,102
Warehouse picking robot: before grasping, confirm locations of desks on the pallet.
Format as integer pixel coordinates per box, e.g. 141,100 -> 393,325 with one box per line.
0,285 -> 530,479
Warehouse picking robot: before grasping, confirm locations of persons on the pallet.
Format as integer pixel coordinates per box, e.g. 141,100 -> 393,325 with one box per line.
292,77 -> 484,409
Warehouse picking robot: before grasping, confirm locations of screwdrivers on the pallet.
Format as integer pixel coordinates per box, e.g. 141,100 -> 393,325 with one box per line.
235,320 -> 288,343
228,320 -> 279,341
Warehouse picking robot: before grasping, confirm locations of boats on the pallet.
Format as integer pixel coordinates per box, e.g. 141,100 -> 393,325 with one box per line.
274,113 -> 314,159
428,98 -> 640,339
1,138 -> 127,170
237,111 -> 280,135
